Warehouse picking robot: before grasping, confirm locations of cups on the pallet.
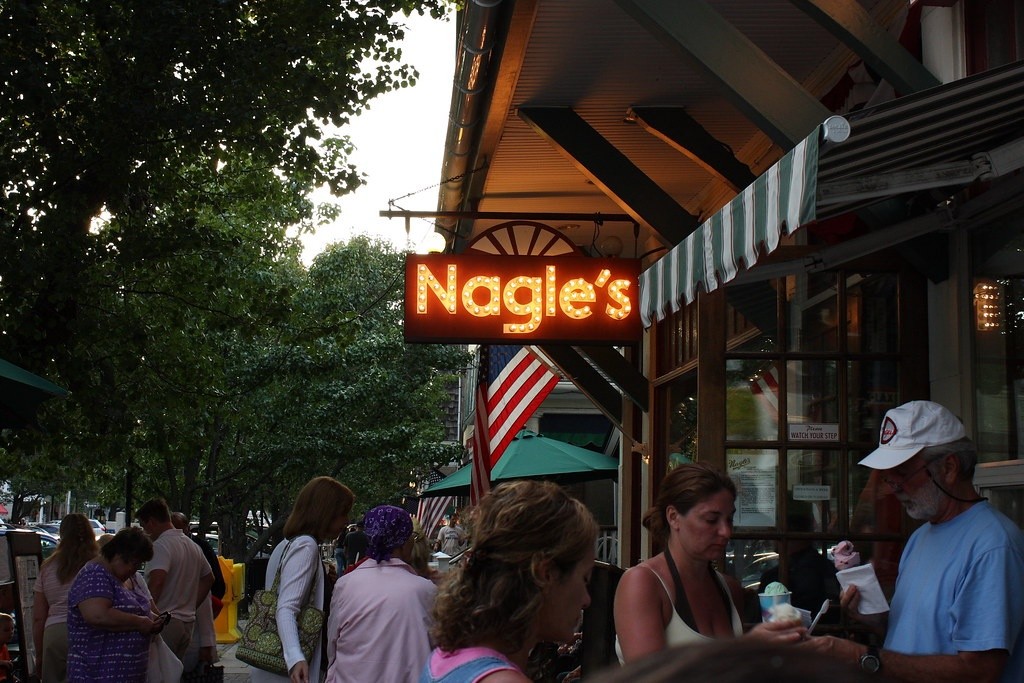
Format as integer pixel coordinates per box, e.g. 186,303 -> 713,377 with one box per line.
757,592 -> 792,623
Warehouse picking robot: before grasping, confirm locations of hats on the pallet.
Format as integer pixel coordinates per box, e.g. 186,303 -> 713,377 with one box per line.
857,400 -> 967,470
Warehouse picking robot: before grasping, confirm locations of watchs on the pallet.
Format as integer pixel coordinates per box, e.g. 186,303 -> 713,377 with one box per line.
860,645 -> 880,674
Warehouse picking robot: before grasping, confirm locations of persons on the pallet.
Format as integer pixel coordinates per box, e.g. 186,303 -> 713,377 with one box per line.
32,496 -> 226,683
0,612 -> 15,683
613,398 -> 1024,683
244,474 -> 470,683
416,479 -> 600,683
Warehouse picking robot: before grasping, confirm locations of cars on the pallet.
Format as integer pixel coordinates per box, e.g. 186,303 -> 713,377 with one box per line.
0,518 -> 111,563
187,510 -> 276,558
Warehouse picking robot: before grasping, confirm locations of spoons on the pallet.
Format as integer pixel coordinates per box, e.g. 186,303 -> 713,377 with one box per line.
800,599 -> 829,641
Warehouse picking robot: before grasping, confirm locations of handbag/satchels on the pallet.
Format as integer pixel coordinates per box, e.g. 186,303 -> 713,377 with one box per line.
146,632 -> 184,683
235,535 -> 325,679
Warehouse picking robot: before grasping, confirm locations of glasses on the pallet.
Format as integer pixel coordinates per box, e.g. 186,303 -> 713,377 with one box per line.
152,611 -> 172,635
412,531 -> 419,539
879,458 -> 939,490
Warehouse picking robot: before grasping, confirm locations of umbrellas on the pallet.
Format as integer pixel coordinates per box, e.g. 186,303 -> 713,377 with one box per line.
418,423 -> 618,495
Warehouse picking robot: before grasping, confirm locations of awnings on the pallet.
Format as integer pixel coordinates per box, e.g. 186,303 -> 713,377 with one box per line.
635,55 -> 1024,331
0,359 -> 71,432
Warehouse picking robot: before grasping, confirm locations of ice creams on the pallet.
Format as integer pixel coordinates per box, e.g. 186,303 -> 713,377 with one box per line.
832,540 -> 861,570
771,602 -> 801,623
763,581 -> 789,595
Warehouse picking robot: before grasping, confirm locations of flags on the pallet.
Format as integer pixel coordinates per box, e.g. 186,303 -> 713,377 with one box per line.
469,346 -> 559,508
422,496 -> 454,538
417,497 -> 429,527
